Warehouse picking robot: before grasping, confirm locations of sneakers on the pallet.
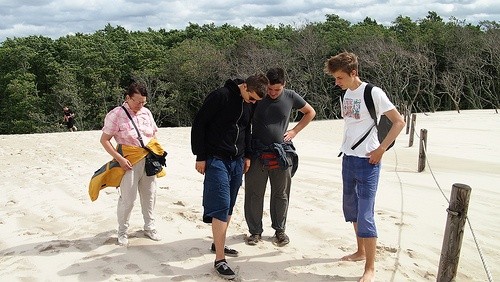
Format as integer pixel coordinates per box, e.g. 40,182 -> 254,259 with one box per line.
248,233 -> 261,244
118,233 -> 128,245
144,229 -> 162,241
211,243 -> 238,256
275,231 -> 289,244
214,259 -> 235,279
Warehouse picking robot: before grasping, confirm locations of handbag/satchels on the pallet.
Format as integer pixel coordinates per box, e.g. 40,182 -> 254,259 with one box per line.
145,148 -> 168,176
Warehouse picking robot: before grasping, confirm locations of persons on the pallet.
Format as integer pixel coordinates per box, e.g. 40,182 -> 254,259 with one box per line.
244,68 -> 317,246
323,50 -> 404,282
62,106 -> 78,132
190,73 -> 269,279
100,82 -> 161,246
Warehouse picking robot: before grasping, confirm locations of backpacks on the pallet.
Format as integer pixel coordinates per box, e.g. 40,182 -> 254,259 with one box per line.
341,80 -> 396,151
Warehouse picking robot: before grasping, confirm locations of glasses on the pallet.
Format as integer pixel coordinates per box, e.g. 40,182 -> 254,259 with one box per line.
130,96 -> 147,105
249,92 -> 261,103
